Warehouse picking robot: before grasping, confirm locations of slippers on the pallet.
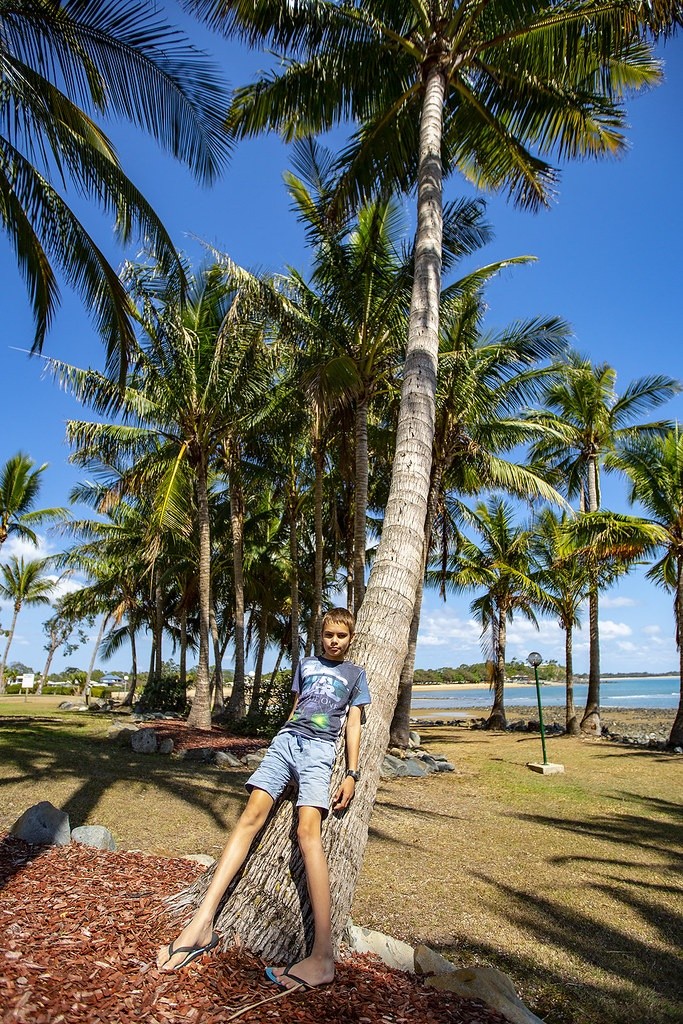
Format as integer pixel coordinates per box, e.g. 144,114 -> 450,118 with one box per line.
158,932 -> 219,973
264,962 -> 333,993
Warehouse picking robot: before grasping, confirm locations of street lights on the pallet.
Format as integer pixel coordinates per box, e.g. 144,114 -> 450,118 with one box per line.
526,652 -> 566,774
123,675 -> 129,699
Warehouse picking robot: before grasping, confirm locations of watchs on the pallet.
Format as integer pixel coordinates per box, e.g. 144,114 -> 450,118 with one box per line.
345,769 -> 360,782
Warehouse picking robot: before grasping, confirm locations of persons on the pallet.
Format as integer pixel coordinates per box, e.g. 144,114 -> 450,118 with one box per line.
155,608 -> 372,993
